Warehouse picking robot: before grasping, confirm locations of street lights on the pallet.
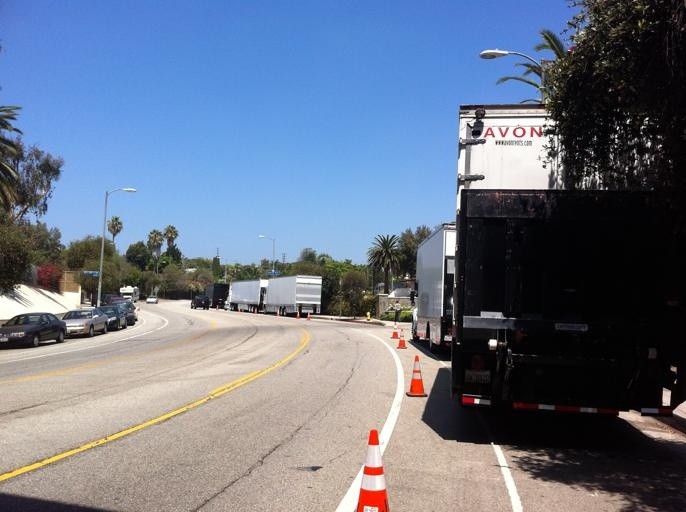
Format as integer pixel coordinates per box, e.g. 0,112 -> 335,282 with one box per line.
479,49 -> 545,76
216,255 -> 227,284
156,261 -> 166,297
96,188 -> 136,308
258,234 -> 276,279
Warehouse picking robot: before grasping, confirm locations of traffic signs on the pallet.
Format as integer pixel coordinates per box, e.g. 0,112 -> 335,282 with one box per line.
83,271 -> 98,276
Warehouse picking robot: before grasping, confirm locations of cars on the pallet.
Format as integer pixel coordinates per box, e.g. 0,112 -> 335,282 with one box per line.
102,294 -> 139,326
145,295 -> 158,303
96,306 -> 127,331
59,308 -> 109,339
0,312 -> 67,348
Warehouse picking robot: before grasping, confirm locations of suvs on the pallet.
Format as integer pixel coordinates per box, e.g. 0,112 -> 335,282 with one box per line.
190,295 -> 209,310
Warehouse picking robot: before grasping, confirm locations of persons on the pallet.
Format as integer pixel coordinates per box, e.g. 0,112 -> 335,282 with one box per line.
387,301 -> 402,326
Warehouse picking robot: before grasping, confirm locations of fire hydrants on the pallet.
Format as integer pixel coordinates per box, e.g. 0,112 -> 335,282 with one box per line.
367,311 -> 370,321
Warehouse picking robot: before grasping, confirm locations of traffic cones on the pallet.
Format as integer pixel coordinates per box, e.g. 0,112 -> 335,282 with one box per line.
406,355 -> 428,397
396,328 -> 407,348
354,428 -> 389,512
305,312 -> 313,322
275,308 -> 281,318
216,305 -> 219,310
237,307 -> 241,313
253,308 -> 257,314
296,311 -> 300,319
391,321 -> 400,339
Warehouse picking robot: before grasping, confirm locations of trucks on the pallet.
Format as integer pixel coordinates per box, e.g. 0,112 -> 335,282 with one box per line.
410,223 -> 457,353
119,284 -> 139,303
202,283 -> 229,308
451,104 -> 685,417
262,274 -> 323,317
226,279 -> 268,313
